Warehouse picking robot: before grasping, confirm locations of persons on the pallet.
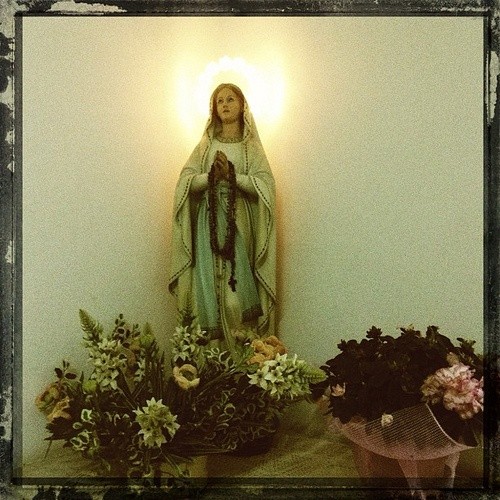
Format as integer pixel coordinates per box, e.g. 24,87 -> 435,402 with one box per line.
168,83 -> 277,344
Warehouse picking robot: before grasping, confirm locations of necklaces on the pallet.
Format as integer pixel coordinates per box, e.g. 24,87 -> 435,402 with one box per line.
208,160 -> 237,292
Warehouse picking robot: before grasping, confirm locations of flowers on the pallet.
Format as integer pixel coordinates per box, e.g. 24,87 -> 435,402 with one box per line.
316,325 -> 487,450
31,307 -> 326,481
